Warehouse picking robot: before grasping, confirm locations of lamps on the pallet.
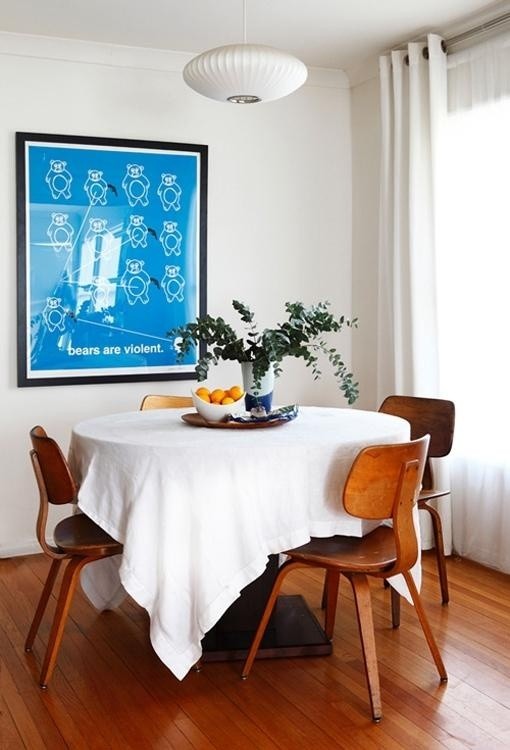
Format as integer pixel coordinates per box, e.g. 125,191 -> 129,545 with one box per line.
182,0 -> 309,105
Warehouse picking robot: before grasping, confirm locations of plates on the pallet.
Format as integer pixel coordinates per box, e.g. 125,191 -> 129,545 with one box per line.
182,414 -> 286,429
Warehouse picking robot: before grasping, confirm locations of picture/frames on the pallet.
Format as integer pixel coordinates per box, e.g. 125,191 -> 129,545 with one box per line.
16,131 -> 208,387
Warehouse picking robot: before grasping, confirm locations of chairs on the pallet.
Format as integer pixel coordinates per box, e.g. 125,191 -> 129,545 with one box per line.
25,425 -> 203,689
141,394 -> 194,411
321,396 -> 454,627
240,433 -> 448,723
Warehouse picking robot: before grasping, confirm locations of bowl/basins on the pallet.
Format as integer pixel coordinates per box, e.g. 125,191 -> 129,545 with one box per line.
191,387 -> 246,424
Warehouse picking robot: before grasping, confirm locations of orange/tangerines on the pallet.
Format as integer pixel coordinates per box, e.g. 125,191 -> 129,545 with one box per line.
197,386 -> 243,406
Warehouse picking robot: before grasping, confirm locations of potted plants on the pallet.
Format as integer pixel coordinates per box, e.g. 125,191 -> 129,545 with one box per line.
166,300 -> 358,412
